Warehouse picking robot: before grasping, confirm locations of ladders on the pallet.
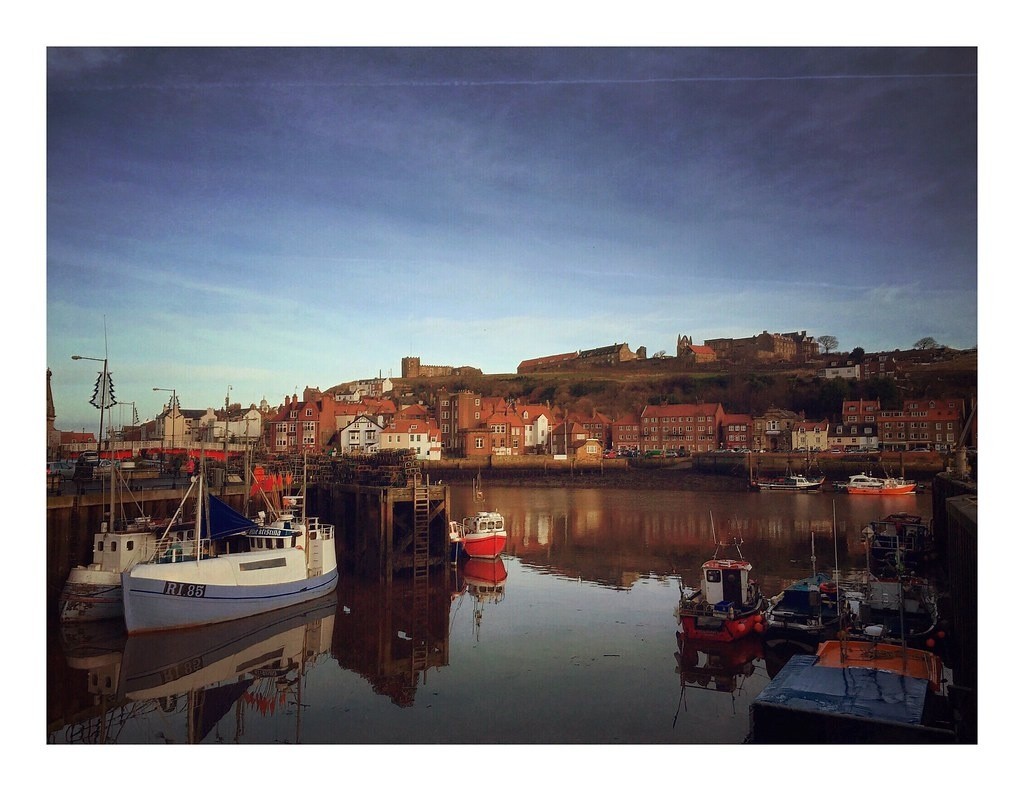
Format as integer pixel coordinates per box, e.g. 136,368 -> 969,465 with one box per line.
413,473 -> 429,578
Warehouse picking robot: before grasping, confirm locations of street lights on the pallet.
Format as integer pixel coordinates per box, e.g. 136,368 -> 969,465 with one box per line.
70,355 -> 118,469
152,388 -> 183,448
115,401 -> 139,449
224,385 -> 233,471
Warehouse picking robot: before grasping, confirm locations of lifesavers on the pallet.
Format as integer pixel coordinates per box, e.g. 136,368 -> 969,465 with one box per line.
885,483 -> 893,489
819,582 -> 839,594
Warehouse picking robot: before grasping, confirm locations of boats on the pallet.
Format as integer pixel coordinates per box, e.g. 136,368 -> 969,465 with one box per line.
858,560 -> 940,643
845,470 -> 917,495
118,589 -> 340,745
461,466 -> 507,559
750,463 -> 822,491
672,510 -> 767,641
860,512 -> 939,570
57,433 -> 268,626
768,530 -> 869,640
120,436 -> 339,634
53,626 -> 124,695
464,558 -> 509,643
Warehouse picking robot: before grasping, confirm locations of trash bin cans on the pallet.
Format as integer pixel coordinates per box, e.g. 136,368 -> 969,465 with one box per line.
75,462 -> 93,478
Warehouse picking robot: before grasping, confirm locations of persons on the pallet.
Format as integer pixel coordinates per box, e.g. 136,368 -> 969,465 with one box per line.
173,458 -> 199,483
727,575 -> 740,601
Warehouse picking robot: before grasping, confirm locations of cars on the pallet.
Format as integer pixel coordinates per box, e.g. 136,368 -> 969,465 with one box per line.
906,447 -> 931,453
713,447 -> 880,454
602,450 -> 689,458
